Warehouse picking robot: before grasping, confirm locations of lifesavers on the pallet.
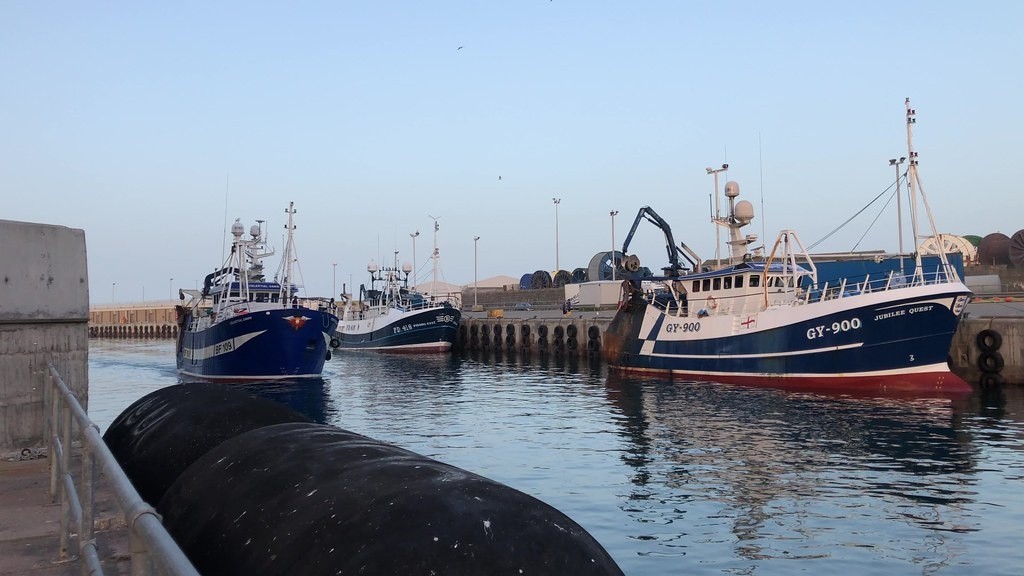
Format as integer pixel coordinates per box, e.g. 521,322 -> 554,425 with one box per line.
521,324 -> 531,351
706,295 -> 718,309
537,325 -> 548,352
587,326 -> 600,359
552,325 -> 564,355
976,329 -> 1006,386
506,323 -> 515,352
325,349 -> 331,361
566,325 -> 578,356
469,324 -> 478,350
481,324 -> 490,352
459,325 -> 467,350
493,324 -> 502,352
330,338 -> 340,349
88,324 -> 177,339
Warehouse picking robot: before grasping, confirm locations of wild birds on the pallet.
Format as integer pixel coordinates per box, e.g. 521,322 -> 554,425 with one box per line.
457,45 -> 463,50
499,176 -> 503,181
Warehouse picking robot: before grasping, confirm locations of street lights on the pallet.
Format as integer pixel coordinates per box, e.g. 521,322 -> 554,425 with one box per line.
472,234 -> 481,312
609,209 -> 620,280
551,197 -> 561,272
409,231 -> 420,288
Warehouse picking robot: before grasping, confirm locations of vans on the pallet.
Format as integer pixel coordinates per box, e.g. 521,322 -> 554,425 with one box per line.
513,302 -> 535,311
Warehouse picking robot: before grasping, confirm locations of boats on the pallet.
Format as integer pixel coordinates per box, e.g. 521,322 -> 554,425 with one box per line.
332,232 -> 463,354
173,159 -> 341,381
602,96 -> 979,400
